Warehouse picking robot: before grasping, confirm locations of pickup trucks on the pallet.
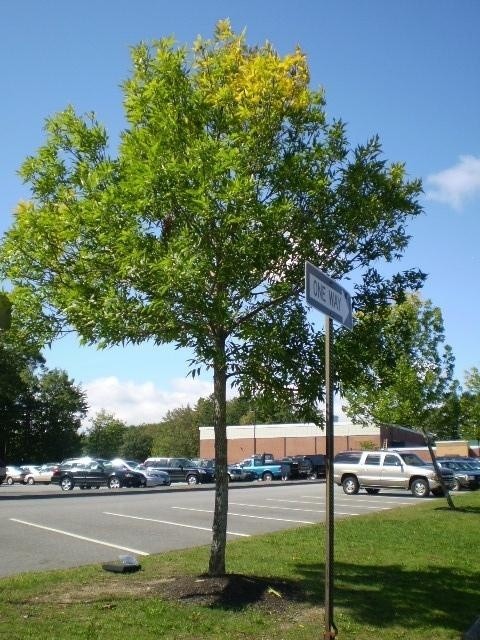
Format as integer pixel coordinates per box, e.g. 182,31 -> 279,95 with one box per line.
333,450 -> 457,498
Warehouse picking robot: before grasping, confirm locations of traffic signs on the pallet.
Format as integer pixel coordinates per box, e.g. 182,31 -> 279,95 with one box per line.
305,260 -> 354,335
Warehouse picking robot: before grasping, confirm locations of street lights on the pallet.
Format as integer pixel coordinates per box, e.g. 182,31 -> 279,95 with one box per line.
250,407 -> 257,437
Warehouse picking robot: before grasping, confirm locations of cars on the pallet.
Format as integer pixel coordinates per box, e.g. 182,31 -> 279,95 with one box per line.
125,454 -> 327,488
0,461 -> 62,486
50,456 -> 147,491
435,455 -> 480,491
